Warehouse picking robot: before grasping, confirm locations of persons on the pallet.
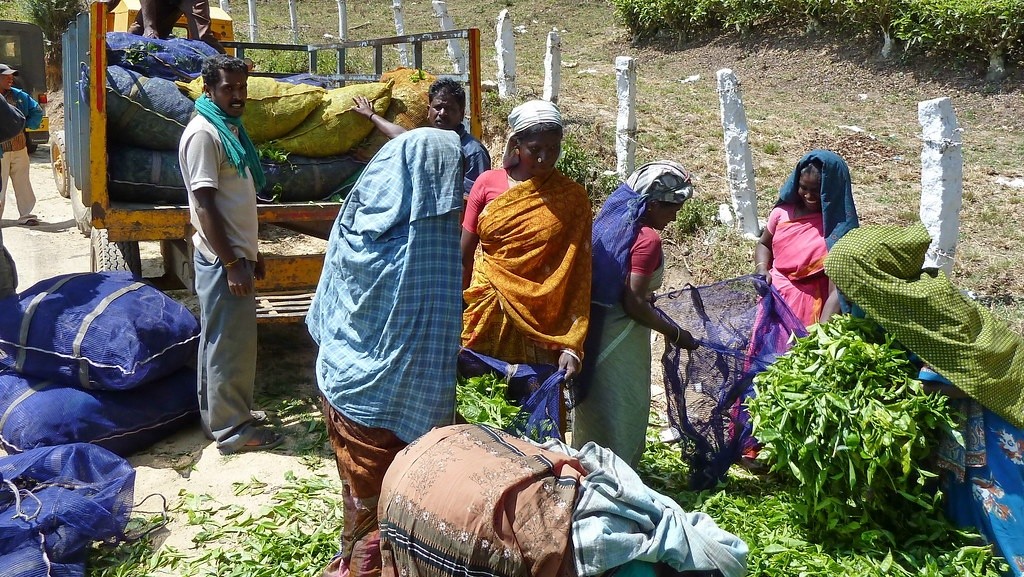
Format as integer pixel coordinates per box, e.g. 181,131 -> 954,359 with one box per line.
731,146 -> 864,457
0,64 -> 40,226
824,221 -> 1024,577
304,79 -> 593,577
178,56 -> 289,454
574,160 -> 694,471
743,452 -> 746,458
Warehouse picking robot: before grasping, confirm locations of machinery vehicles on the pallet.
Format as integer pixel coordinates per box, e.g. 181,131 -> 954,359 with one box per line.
49,0 -> 484,334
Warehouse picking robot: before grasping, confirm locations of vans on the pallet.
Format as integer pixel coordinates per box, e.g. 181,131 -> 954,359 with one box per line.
0,19 -> 50,152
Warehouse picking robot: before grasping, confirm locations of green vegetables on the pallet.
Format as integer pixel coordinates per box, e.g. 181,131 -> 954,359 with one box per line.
254,141 -> 291,202
624,313 -> 1015,577
448,366 -> 554,444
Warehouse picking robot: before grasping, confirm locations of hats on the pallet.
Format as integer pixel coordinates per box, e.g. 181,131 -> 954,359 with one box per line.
0,64 -> 18,74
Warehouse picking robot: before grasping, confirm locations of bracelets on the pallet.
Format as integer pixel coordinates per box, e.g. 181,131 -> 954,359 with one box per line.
223,256 -> 240,269
673,325 -> 681,344
755,262 -> 770,271
369,112 -> 377,121
559,349 -> 583,375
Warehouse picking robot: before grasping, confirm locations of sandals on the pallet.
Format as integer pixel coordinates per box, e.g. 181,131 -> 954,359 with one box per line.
251,409 -> 282,427
237,426 -> 285,451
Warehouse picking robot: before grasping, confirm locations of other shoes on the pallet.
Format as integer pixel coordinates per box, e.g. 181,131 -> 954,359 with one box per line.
680,477 -> 718,491
639,475 -> 667,493
17,219 -> 40,227
751,454 -> 778,475
